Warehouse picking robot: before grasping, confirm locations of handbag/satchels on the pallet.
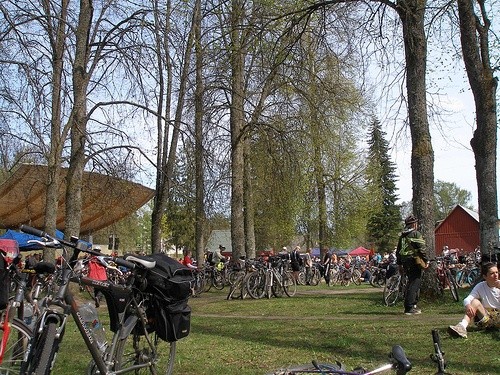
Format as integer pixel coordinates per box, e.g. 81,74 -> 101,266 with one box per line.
108,253 -> 196,343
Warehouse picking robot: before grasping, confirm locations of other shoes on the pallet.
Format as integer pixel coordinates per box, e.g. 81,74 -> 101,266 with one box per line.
403,308 -> 421,315
414,304 -> 421,314
192,295 -> 198,298
306,281 -> 311,285
94,296 -> 100,308
296,279 -> 300,285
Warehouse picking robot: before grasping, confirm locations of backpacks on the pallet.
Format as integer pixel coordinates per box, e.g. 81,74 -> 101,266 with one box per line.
206,251 -> 213,262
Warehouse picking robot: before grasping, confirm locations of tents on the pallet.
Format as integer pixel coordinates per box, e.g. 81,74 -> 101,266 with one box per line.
334,249 -> 348,256
349,246 -> 373,255
311,247 -> 332,257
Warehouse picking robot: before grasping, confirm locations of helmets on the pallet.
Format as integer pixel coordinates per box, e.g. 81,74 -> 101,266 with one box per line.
93,245 -> 102,250
219,244 -> 226,249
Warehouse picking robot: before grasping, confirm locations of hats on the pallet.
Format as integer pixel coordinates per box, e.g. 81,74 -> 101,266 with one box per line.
405,216 -> 418,224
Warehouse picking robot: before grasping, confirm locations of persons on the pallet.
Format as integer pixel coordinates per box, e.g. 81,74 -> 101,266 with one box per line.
182,250 -> 200,299
0,252 -> 92,276
396,216 -> 430,316
260,245 -> 407,288
82,248 -> 109,308
447,261 -> 500,341
209,244 -> 226,288
178,249 -> 233,267
439,244 -> 482,269
105,250 -> 147,258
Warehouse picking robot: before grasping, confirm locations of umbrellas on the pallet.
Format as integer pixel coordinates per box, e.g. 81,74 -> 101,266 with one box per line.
54,228 -> 93,252
0,228 -> 45,252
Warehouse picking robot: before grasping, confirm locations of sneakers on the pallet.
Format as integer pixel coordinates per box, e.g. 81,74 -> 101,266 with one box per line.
447,324 -> 468,339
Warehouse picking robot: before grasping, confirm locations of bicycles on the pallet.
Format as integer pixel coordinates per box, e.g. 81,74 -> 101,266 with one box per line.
21,225 -> 177,375
265,329 -> 450,375
190,252 -> 482,309
0,249 -> 62,375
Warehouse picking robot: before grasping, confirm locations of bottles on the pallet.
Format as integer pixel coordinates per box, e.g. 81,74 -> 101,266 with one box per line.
20,299 -> 34,328
76,302 -> 109,347
